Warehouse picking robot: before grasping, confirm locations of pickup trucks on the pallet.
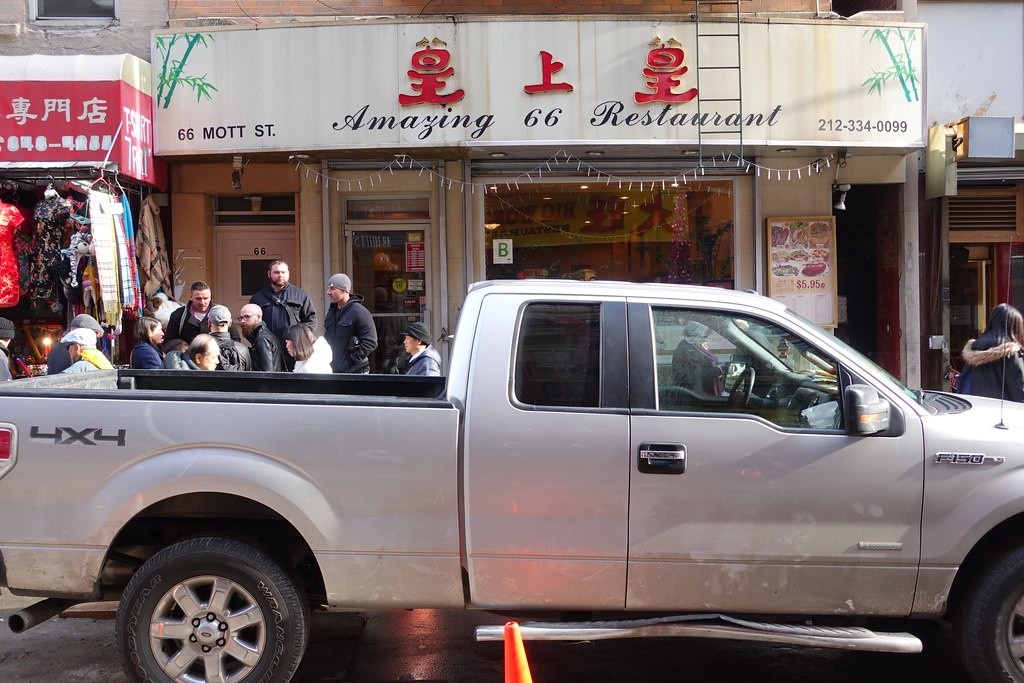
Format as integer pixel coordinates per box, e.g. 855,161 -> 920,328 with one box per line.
0,277 -> 1024,683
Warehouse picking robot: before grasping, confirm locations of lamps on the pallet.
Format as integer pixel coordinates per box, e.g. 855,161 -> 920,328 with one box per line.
834,183 -> 851,210
231,168 -> 245,193
250,196 -> 262,215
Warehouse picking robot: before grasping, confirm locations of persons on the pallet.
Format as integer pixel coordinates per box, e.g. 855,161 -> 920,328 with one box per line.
399,321 -> 441,376
249,261 -> 317,372
323,273 -> 378,374
729,319 -> 770,362
158,334 -> 220,370
0,200 -> 25,307
129,316 -> 189,369
956,303 -> 1024,403
671,321 -> 722,396
0,317 -> 15,381
283,324 -> 333,374
60,328 -> 114,373
206,305 -> 252,371
30,189 -> 72,299
238,304 -> 282,372
47,314 -> 104,374
163,281 -> 242,344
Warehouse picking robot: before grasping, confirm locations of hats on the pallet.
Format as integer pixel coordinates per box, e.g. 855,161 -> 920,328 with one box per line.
71,314 -> 104,333
328,274 -> 351,293
60,328 -> 96,346
371,287 -> 387,301
0,317 -> 15,339
207,305 -> 231,326
398,322 -> 430,345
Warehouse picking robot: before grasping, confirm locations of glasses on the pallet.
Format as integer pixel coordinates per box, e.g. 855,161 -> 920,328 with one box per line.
66,343 -> 81,350
238,314 -> 260,322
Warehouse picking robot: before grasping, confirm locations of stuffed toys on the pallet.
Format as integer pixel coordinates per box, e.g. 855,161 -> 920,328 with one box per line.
69,231 -> 95,287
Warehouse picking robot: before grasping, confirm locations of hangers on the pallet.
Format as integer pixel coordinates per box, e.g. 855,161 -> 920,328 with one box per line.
88,168 -> 125,198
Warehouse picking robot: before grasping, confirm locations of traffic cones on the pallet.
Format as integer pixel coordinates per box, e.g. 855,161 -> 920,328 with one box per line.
504,621 -> 533,683
